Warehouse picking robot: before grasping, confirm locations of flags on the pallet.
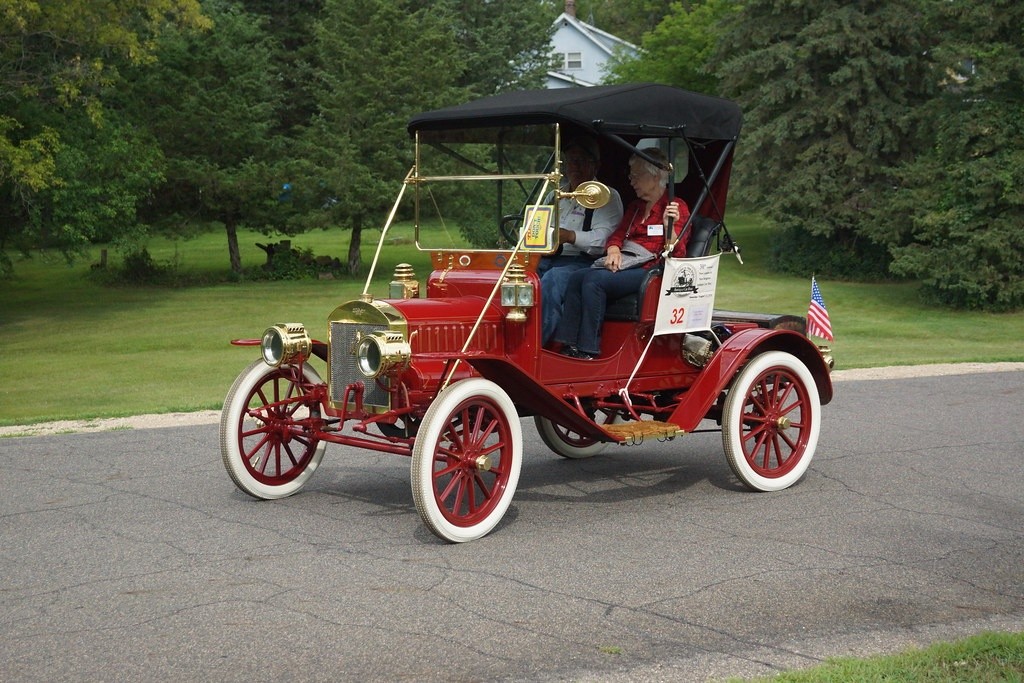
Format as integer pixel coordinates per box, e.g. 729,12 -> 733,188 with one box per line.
807,279 -> 834,340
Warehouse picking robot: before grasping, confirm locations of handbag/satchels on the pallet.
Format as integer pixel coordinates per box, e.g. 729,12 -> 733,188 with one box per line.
590,239 -> 656,270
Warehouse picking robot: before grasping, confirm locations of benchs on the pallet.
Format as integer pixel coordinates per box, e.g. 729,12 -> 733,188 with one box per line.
604,217 -> 723,322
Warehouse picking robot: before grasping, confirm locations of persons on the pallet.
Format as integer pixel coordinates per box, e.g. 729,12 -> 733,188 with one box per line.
541,135 -> 624,346
553,147 -> 692,359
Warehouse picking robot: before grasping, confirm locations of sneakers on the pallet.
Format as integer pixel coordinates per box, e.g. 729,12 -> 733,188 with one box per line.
558,344 -> 593,360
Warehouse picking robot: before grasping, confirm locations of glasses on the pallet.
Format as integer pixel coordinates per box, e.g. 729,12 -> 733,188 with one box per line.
628,171 -> 649,180
